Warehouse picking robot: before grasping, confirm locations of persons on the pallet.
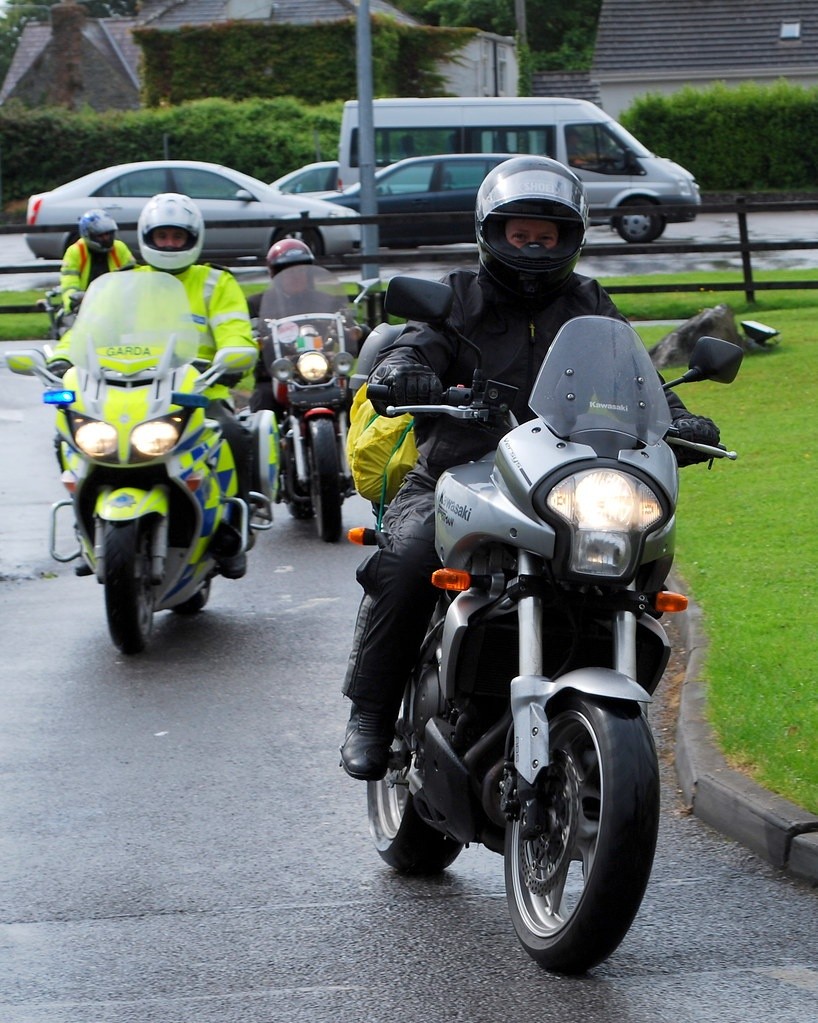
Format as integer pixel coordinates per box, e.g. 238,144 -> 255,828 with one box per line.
565,128 -> 604,166
248,238 -> 372,425
341,156 -> 720,782
59,209 -> 136,328
45,192 -> 260,580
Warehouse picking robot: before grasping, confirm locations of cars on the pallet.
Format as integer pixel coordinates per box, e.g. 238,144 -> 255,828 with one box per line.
321,153 -> 532,244
24,162 -> 366,270
272,162 -> 350,212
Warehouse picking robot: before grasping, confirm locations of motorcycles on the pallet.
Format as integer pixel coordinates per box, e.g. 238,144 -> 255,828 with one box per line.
2,341 -> 282,656
337,274 -> 745,976
248,262 -> 372,542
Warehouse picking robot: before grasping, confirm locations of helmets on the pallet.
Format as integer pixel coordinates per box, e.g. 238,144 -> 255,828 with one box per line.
475,155 -> 588,301
79,209 -> 118,253
137,193 -> 205,270
267,238 -> 315,286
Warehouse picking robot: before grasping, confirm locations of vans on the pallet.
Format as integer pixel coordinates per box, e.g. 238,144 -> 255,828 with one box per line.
341,94 -> 701,242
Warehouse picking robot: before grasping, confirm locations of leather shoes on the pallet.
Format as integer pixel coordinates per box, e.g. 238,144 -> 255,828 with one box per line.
219,552 -> 246,579
76,560 -> 93,576
342,701 -> 397,780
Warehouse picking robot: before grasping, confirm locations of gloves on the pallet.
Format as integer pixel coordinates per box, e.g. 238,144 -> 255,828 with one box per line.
673,417 -> 721,468
206,363 -> 243,388
44,360 -> 72,384
380,364 -> 441,418
